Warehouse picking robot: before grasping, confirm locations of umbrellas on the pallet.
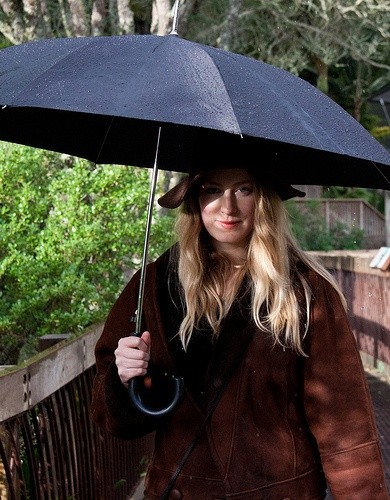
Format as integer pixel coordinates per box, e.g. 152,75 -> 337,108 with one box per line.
0,0 -> 390,422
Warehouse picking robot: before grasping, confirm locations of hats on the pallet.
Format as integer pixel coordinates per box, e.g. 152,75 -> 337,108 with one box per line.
157,132 -> 306,209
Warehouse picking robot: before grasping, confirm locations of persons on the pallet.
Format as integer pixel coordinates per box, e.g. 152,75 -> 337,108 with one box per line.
87,123 -> 388,500
